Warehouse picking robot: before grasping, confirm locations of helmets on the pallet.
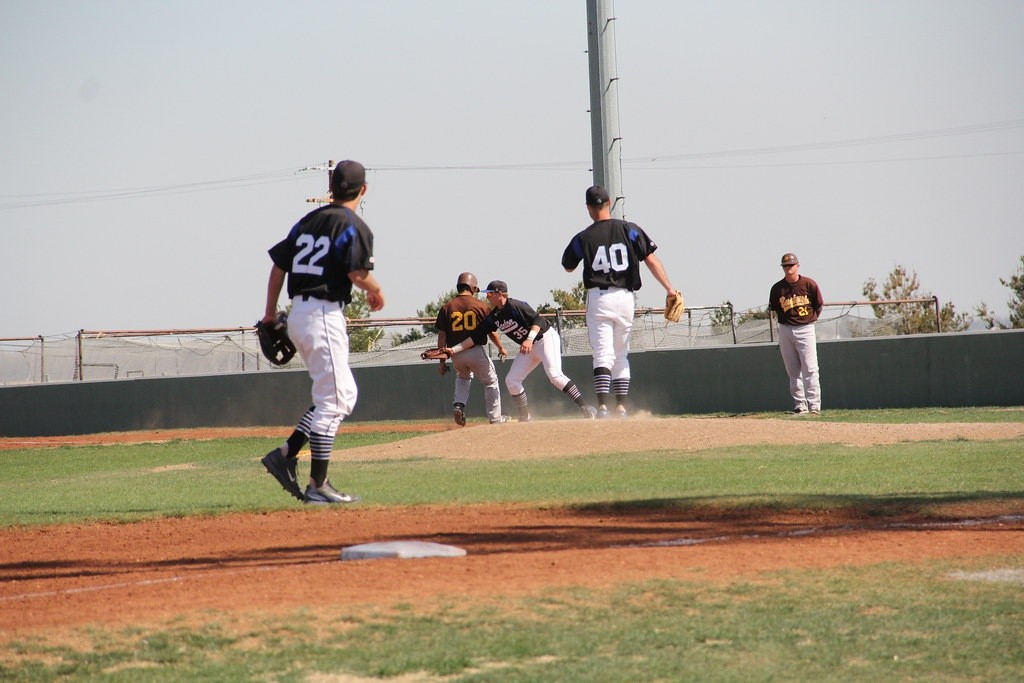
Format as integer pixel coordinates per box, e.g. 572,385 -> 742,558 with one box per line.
456,272 -> 478,294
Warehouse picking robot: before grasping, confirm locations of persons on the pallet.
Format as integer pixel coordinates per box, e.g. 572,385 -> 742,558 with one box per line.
562,185 -> 685,419
420,280 -> 597,422
254,161 -> 384,503
435,272 -> 513,427
768,253 -> 823,415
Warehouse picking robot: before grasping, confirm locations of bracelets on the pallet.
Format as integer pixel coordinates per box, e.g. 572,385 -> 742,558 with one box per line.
451,342 -> 464,354
526,329 -> 537,342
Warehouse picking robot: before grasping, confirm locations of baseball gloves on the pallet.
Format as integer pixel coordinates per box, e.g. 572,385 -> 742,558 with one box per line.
420,348 -> 453,361
663,289 -> 685,328
438,358 -> 451,376
251,303 -> 296,366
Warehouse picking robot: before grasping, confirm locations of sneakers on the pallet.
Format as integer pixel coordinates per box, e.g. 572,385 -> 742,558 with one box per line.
262,447 -> 304,501
303,480 -> 358,505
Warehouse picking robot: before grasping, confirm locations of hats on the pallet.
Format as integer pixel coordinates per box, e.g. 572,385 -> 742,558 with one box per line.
586,186 -> 609,205
480,279 -> 507,294
780,253 -> 799,266
332,161 -> 367,192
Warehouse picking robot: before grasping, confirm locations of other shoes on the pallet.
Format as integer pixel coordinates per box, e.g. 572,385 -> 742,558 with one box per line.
596,406 -> 610,418
452,404 -> 466,426
519,412 -> 533,421
617,407 -> 627,418
580,406 -> 594,419
490,416 -> 511,424
785,406 -> 819,416
